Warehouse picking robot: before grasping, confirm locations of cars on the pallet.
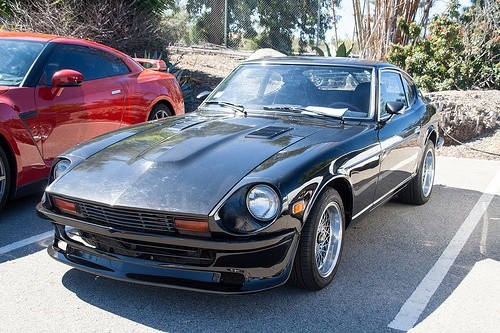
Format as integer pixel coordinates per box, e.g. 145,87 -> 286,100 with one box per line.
34,56 -> 445,296
0,30 -> 187,223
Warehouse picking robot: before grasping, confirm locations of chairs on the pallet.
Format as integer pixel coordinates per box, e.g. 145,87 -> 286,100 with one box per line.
350,82 -> 387,112
273,73 -> 310,107
